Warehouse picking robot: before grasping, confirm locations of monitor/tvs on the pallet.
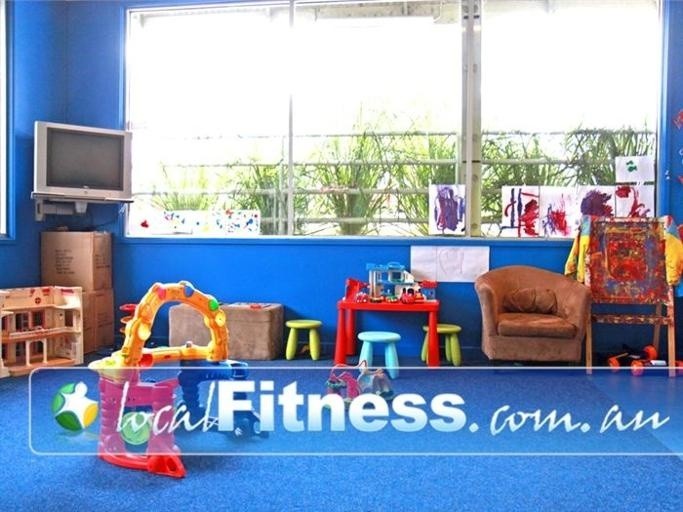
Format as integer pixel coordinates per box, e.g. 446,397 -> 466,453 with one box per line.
34,121 -> 132,198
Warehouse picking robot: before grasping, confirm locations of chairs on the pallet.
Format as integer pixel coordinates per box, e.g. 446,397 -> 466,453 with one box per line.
471,261 -> 591,365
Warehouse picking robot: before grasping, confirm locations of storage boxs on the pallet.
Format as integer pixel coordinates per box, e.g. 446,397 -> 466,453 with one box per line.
76,291 -> 98,353
40,228 -> 114,290
92,284 -> 118,349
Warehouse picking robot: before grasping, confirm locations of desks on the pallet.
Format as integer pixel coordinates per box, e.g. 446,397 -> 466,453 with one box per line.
331,297 -> 442,373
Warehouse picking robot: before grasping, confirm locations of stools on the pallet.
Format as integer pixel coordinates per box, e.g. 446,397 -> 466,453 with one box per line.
161,300 -> 283,364
354,331 -> 403,383
281,319 -> 323,364
419,319 -> 464,368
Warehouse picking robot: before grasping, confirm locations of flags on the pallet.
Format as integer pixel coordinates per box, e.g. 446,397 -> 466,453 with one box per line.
323,360 -> 398,409
82,279 -> 271,479
338,260 -> 438,304
605,341 -> 683,376
